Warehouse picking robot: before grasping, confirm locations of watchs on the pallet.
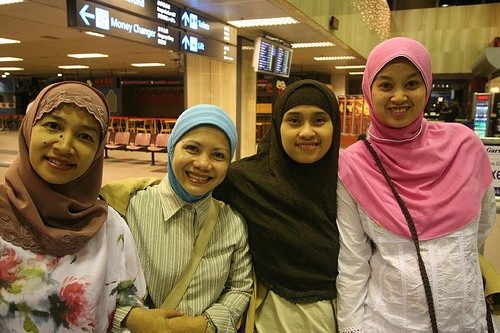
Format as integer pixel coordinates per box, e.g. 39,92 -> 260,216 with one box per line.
202,312 -> 217,333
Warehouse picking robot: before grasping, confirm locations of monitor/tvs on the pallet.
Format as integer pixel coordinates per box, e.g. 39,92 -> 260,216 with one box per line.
255,38 -> 292,77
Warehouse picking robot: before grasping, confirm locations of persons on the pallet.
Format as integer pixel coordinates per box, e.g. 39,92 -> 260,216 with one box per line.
336,37 -> 497,333
98,79 -> 500,333
110,104 -> 253,333
0,81 -> 146,333
438,100 -> 464,122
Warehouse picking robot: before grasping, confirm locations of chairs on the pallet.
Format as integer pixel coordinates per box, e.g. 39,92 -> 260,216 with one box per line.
104,117 -> 177,165
